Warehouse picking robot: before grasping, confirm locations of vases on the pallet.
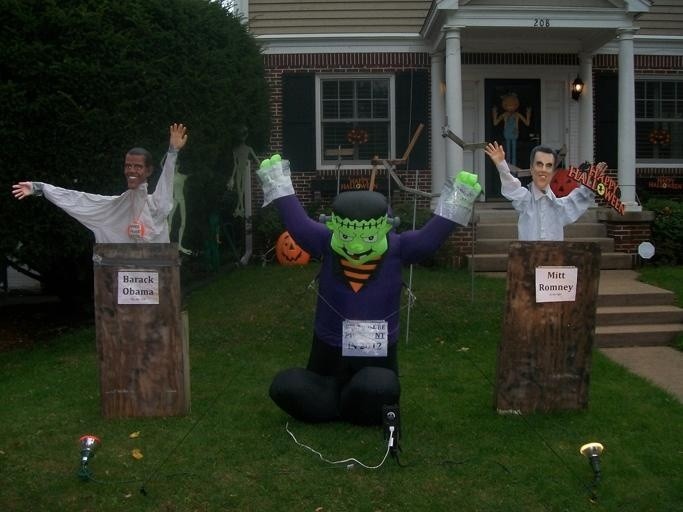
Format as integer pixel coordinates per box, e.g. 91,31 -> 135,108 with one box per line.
353,143 -> 359,160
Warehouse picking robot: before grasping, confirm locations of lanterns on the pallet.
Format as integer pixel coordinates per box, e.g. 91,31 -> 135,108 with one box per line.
549,169 -> 580,197
277,229 -> 309,266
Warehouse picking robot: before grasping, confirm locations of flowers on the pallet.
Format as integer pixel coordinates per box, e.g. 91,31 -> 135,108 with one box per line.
347,129 -> 368,145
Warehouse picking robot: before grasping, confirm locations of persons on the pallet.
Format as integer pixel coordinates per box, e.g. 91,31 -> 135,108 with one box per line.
11,122 -> 188,243
485,139 -> 611,242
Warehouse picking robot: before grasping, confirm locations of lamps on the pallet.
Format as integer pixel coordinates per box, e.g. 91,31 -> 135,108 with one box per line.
580,442 -> 604,488
78,435 -> 102,480
572,74 -> 585,101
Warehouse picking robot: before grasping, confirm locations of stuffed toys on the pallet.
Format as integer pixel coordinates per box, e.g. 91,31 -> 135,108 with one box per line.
254,150 -> 485,425
